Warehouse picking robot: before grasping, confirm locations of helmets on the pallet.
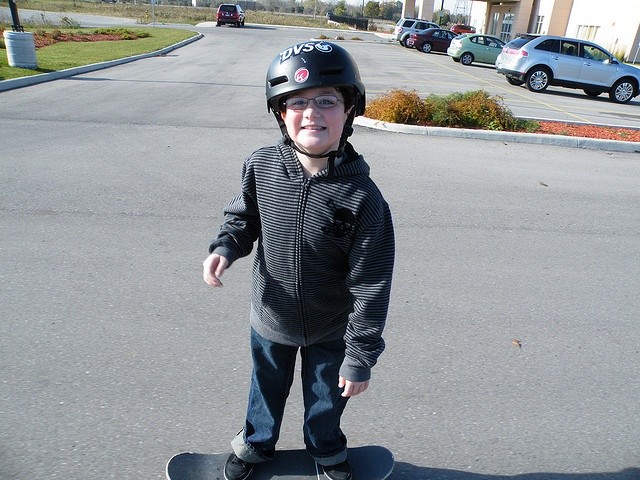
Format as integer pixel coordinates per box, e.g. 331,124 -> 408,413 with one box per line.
266,41 -> 366,117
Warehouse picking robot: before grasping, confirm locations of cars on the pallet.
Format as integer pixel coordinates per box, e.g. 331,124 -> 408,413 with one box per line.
447,33 -> 506,65
409,28 -> 459,53
450,25 -> 476,35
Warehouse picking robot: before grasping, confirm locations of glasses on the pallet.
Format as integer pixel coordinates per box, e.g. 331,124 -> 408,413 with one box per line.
282,95 -> 346,110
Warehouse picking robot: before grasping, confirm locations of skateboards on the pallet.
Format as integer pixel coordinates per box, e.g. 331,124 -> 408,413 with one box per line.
167,445 -> 394,479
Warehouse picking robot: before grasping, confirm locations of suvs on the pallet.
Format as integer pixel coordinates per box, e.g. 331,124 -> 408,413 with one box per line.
495,33 -> 640,104
216,4 -> 245,27
393,19 -> 442,48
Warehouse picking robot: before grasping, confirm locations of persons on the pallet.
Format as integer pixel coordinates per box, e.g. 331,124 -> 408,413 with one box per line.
201,41 -> 397,479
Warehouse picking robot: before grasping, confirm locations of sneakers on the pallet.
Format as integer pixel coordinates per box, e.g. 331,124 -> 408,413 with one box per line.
321,457 -> 353,480
223,450 -> 256,480
